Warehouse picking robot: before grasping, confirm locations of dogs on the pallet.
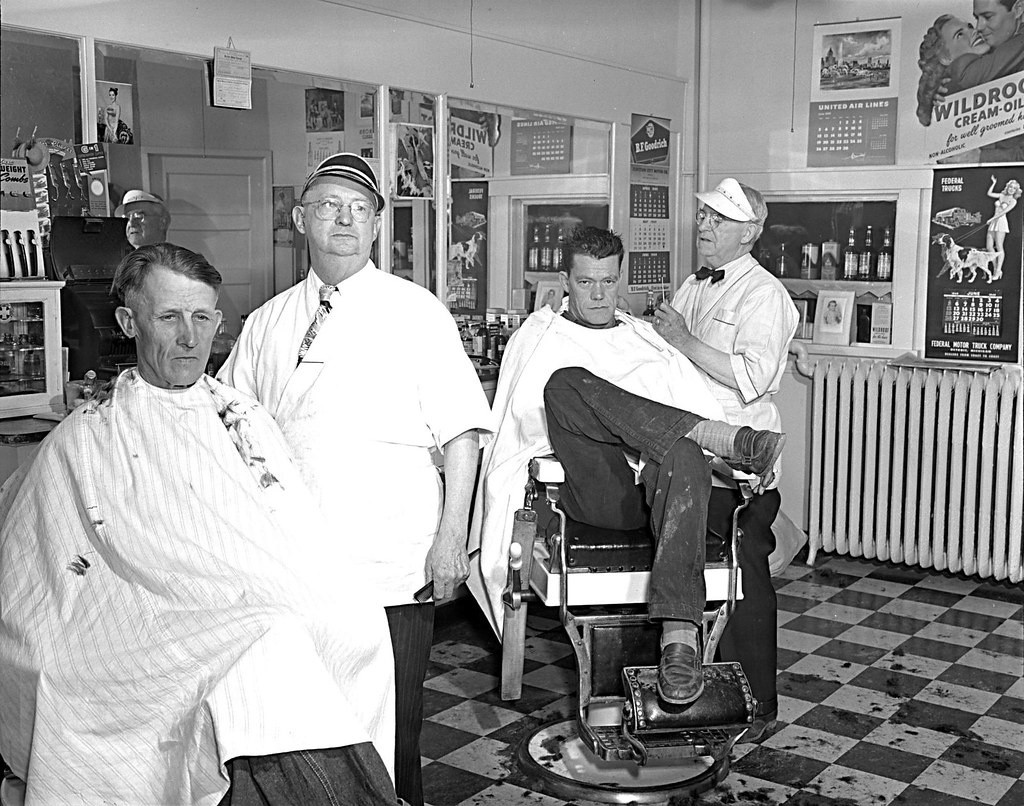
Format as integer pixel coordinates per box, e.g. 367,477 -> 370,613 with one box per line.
451,232 -> 485,269
932,233 -> 1003,285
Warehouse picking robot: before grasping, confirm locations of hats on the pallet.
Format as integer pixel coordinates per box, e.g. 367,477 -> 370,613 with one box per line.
299,152 -> 386,214
693,176 -> 765,228
113,189 -> 170,218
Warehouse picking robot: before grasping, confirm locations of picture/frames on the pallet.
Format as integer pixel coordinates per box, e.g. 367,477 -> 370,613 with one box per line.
533,281 -> 565,311
811,291 -> 856,348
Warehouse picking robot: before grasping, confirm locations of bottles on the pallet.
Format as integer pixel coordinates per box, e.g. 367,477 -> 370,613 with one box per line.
876,225 -> 893,282
859,225 -> 875,281
407,227 -> 413,269
553,228 -> 568,272
206,313 -> 249,377
842,225 -> 858,281
457,316 -> 513,364
643,289 -> 655,317
858,308 -> 870,342
528,225 -> 540,271
540,225 -> 552,272
775,242 -> 790,277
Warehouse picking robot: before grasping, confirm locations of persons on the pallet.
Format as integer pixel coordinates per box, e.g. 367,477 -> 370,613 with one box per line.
823,301 -> 841,325
307,99 -> 343,131
1,244 -> 401,806
617,177 -> 800,741
276,191 -> 291,225
104,88 -> 121,142
986,175 -> 1022,280
114,190 -> 172,248
214,153 -> 497,806
545,290 -> 555,309
508,227 -> 787,704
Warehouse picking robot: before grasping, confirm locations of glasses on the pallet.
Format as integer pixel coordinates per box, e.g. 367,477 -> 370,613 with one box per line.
120,211 -> 164,226
694,208 -> 746,231
307,197 -> 379,224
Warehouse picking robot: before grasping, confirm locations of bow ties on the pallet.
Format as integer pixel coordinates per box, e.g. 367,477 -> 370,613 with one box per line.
694,265 -> 725,284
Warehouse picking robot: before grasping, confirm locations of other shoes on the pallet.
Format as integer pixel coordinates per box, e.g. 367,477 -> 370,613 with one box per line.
737,716 -> 778,743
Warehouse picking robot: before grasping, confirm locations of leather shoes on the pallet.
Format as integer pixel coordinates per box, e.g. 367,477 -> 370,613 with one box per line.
657,629 -> 704,706
724,425 -> 787,477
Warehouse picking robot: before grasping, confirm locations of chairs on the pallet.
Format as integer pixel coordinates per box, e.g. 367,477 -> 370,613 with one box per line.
496,457 -> 746,702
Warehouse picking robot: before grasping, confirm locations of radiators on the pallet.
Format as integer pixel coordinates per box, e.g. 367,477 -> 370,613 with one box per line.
805,362 -> 1024,588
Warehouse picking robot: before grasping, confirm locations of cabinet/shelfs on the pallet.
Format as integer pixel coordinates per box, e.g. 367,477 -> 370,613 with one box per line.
749,192 -> 901,344
0,279 -> 67,419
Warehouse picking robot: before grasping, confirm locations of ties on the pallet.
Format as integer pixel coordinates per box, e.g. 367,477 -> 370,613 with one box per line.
296,285 -> 336,368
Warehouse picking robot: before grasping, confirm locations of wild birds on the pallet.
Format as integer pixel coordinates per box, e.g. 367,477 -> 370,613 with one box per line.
399,125 -> 433,197
